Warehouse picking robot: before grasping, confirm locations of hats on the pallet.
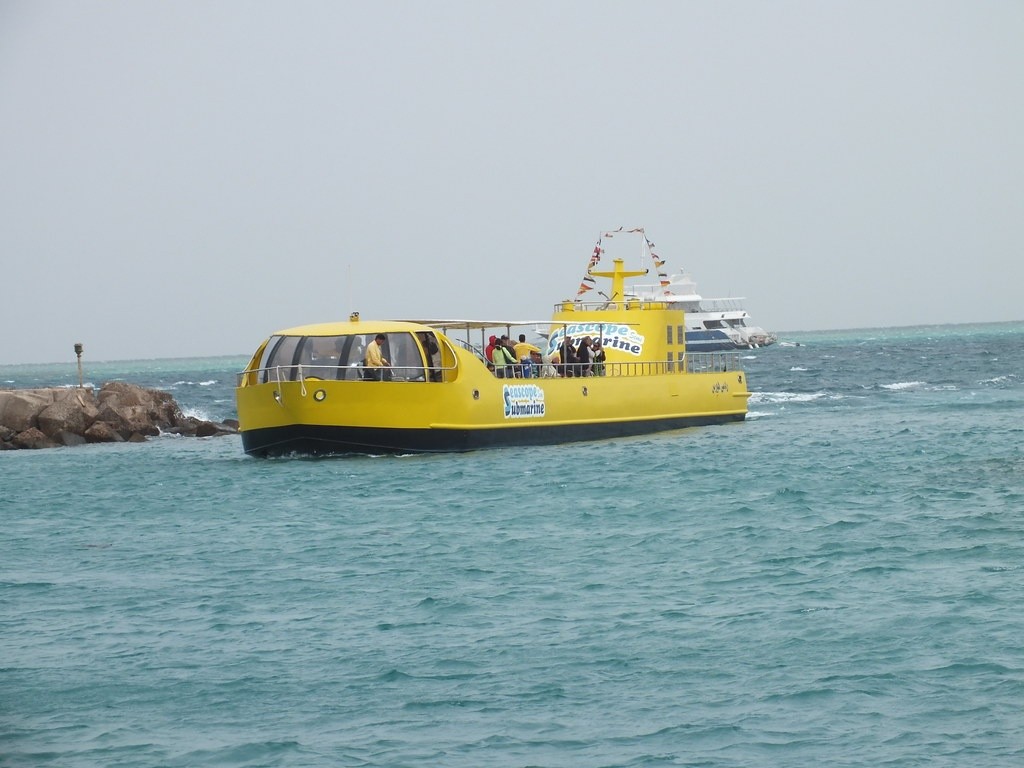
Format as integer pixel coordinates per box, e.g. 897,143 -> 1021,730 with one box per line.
501,335 -> 509,340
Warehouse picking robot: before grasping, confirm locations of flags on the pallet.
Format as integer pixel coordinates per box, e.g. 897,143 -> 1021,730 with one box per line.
577,226 -> 671,296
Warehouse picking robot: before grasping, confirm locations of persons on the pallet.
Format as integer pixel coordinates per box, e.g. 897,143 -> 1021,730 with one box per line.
364,334 -> 391,381
486,334 -> 542,379
551,336 -> 606,378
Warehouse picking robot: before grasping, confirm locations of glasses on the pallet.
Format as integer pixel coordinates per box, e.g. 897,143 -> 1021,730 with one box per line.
594,345 -> 598,347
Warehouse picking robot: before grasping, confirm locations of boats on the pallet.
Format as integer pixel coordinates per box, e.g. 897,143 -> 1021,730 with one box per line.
533,269 -> 779,351
238,226 -> 752,458
